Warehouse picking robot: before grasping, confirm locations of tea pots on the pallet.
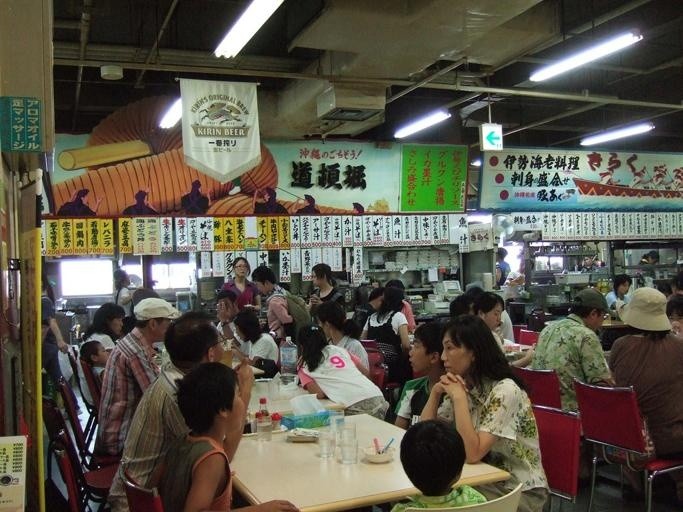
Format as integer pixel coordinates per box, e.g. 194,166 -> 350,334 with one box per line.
482,272 -> 496,291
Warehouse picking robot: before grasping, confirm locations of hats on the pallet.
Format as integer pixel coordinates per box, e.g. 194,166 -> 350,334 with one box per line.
616,287 -> 672,332
573,288 -> 618,318
385,287 -> 404,300
133,298 -> 182,322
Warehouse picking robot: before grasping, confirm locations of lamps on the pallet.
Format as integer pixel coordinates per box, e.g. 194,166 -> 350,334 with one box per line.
155,97 -> 186,132
577,118 -> 656,148
210,0 -> 285,60
393,106 -> 451,141
527,27 -> 643,85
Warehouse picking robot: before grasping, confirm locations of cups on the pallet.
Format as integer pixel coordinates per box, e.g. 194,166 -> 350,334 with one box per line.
569,294 -> 574,301
266,380 -> 280,399
222,349 -> 233,367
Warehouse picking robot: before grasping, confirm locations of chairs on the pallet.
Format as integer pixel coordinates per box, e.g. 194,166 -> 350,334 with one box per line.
513,324 -> 542,348
571,377 -> 683,510
79,355 -> 105,420
54,432 -> 87,511
530,405 -> 583,509
115,463 -> 164,509
43,403 -> 118,511
367,354 -> 384,387
52,376 -> 121,498
519,366 -> 627,511
66,344 -> 99,451
358,339 -> 402,422
402,482 -> 523,511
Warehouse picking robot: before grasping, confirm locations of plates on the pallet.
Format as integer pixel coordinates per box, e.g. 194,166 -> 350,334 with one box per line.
395,247 -> 457,268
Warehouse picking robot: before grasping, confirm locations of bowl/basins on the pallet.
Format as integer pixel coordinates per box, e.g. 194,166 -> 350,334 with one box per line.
360,445 -> 397,464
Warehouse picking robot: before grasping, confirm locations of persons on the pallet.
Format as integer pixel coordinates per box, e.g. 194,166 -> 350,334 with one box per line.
41,257 -> 298,511
296,248 -> 551,511
528,251 -> 683,460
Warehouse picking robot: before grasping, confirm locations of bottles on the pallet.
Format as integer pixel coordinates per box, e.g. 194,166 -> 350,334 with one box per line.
244,410 -> 280,434
559,292 -> 566,303
256,396 -> 272,441
279,335 -> 298,385
318,409 -> 358,465
597,278 -> 614,293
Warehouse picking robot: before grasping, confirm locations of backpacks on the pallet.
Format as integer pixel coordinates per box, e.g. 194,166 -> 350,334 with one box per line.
266,288 -> 312,327
